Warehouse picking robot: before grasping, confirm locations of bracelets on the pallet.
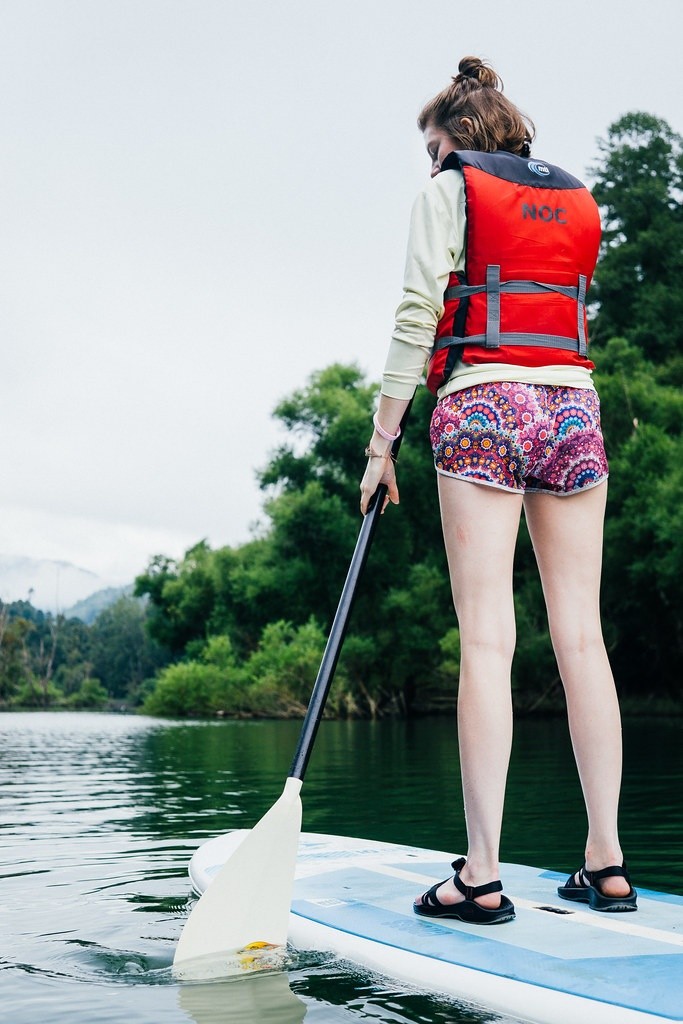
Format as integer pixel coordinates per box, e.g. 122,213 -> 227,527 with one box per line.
373,411 -> 401,440
363,446 -> 398,463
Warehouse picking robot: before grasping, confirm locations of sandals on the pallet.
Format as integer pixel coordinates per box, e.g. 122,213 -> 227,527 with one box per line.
556,862 -> 639,912
412,856 -> 516,925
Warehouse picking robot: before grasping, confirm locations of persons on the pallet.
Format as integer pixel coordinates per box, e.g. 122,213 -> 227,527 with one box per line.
359,54 -> 638,926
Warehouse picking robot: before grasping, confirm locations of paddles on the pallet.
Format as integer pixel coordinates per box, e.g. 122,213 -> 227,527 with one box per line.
169,397 -> 420,974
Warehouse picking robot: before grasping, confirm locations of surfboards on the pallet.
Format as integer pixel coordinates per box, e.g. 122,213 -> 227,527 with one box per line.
186,825 -> 682,1024
